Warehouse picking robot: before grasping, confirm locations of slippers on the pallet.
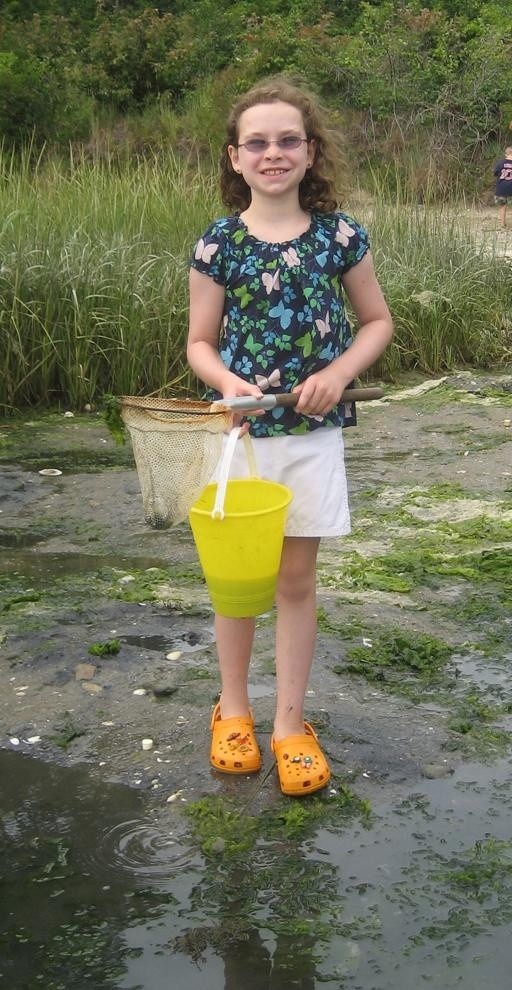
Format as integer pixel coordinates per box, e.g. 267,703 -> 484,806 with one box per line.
210,702 -> 262,775
271,720 -> 330,797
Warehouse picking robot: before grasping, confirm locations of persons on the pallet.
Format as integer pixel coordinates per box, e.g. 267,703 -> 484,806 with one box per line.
492,146 -> 512,227
186,82 -> 395,797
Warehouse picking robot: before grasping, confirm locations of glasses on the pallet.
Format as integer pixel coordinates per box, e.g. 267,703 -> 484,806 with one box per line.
236,136 -> 309,153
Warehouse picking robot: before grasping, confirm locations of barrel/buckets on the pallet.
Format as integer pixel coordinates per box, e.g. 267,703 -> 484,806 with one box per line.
186,426 -> 294,619
